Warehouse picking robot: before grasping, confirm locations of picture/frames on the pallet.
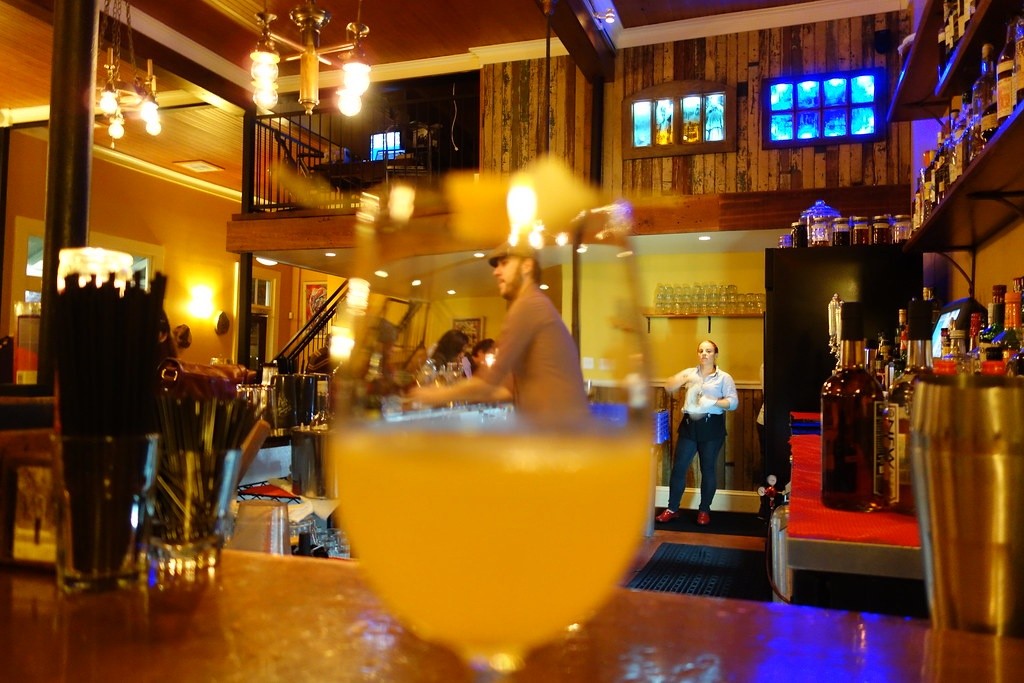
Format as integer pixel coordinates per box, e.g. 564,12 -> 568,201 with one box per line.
300,280 -> 332,336
451,316 -> 489,351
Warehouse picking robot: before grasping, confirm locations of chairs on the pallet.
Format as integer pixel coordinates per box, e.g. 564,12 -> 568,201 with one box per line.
382,122 -> 432,187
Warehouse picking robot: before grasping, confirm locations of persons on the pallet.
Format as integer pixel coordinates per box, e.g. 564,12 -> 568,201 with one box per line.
431,330 -> 496,378
411,243 -> 589,424
656,340 -> 739,524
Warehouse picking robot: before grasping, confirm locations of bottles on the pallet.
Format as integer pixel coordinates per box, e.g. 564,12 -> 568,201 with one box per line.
789,0 -> 1024,247
821,276 -> 1024,508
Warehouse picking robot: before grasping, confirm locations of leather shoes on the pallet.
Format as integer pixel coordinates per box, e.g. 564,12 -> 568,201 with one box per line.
697,511 -> 710,525
655,508 -> 680,522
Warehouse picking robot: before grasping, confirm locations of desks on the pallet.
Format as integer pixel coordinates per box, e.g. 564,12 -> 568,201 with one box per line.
0,552 -> 1024,683
311,155 -> 428,210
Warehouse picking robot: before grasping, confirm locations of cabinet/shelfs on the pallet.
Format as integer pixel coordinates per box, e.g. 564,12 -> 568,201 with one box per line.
885,0 -> 1024,297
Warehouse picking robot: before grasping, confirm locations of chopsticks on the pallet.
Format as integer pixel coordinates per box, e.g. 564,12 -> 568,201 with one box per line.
48,269 -> 167,572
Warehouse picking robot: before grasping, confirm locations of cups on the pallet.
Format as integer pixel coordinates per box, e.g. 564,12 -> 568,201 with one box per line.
322,176 -> 659,683
233,364 -> 328,434
911,372 -> 1024,635
144,448 -> 244,571
51,432 -> 157,592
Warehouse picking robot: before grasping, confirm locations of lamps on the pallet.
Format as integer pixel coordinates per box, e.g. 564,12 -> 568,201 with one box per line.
99,0 -> 161,140
250,0 -> 370,115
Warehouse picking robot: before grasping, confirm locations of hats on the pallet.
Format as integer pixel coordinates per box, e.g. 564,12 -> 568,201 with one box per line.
486,241 -> 541,267
